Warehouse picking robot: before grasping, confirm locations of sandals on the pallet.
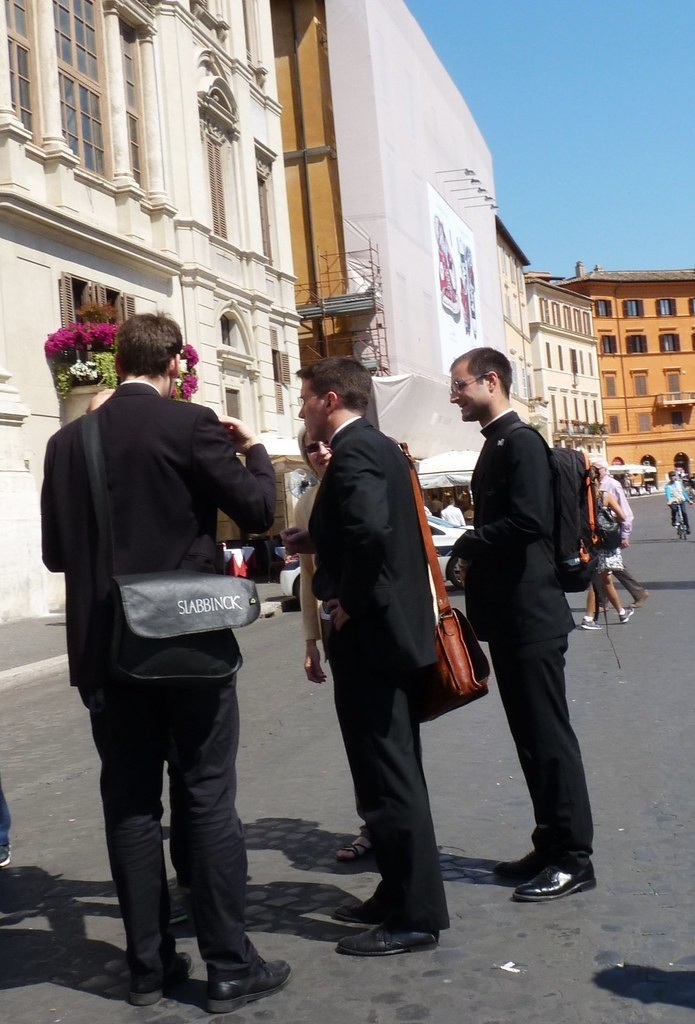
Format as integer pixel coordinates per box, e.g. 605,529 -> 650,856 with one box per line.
334,835 -> 372,860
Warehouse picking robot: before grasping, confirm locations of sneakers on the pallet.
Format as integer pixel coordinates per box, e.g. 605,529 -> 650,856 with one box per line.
580,618 -> 602,630
618,607 -> 634,622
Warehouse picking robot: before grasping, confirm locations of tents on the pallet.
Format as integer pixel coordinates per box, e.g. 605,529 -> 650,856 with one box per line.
416,448 -> 480,490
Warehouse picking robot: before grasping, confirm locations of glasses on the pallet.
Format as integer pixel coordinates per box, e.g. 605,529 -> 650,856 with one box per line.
304,440 -> 331,453
449,371 -> 499,397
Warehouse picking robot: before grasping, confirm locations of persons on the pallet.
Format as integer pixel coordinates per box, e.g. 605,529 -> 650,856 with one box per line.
440,497 -> 467,526
41,310 -> 291,1014
688,472 -> 695,490
296,432 -> 373,861
624,474 -> 631,487
279,356 -> 452,957
593,463 -> 649,612
450,347 -> 598,903
580,467 -> 634,630
665,470 -> 695,535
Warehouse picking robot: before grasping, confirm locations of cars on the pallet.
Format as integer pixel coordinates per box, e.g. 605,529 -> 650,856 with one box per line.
279,517 -> 475,610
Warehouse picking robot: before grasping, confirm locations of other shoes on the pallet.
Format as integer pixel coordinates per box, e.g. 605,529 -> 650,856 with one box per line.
630,589 -> 649,608
672,520 -> 676,526
599,601 -> 610,612
686,530 -> 691,534
0,844 -> 11,866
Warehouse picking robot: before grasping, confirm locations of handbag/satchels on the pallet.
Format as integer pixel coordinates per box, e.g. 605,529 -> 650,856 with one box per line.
600,521 -> 621,548
414,606 -> 491,723
111,570 -> 261,680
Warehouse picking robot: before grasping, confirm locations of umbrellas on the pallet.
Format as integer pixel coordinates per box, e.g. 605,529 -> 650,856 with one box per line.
608,464 -> 657,474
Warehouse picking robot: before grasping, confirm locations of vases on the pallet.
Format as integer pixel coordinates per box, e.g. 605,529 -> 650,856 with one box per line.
92,341 -> 104,349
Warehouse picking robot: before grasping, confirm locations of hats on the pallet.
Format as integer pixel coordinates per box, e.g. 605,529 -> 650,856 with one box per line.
669,472 -> 675,476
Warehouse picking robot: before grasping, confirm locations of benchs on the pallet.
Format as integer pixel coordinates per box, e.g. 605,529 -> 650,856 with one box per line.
215,539 -> 284,583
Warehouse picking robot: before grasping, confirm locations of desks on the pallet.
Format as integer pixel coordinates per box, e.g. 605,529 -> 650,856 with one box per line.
224,546 -> 255,580
272,546 -> 298,575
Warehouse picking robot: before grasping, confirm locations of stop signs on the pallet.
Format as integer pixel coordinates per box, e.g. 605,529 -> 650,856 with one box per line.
613,458 -> 622,467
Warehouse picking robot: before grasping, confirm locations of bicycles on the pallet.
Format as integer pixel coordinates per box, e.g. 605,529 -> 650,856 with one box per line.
669,500 -> 689,540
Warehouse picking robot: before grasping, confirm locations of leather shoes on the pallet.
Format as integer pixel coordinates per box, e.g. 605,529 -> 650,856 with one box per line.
206,955 -> 292,1012
504,849 -> 550,880
337,923 -> 436,955
333,896 -> 385,923
129,952 -> 190,1004
513,859 -> 597,900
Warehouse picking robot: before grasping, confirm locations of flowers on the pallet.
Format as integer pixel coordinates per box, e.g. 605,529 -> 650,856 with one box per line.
44,298 -> 201,401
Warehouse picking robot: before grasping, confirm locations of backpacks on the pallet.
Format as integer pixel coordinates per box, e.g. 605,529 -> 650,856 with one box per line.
498,421 -> 597,594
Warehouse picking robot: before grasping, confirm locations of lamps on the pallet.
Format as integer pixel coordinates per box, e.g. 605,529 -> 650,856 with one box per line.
436,168 -> 499,211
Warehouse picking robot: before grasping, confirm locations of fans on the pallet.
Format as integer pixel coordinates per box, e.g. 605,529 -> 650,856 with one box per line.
289,468 -> 319,499
